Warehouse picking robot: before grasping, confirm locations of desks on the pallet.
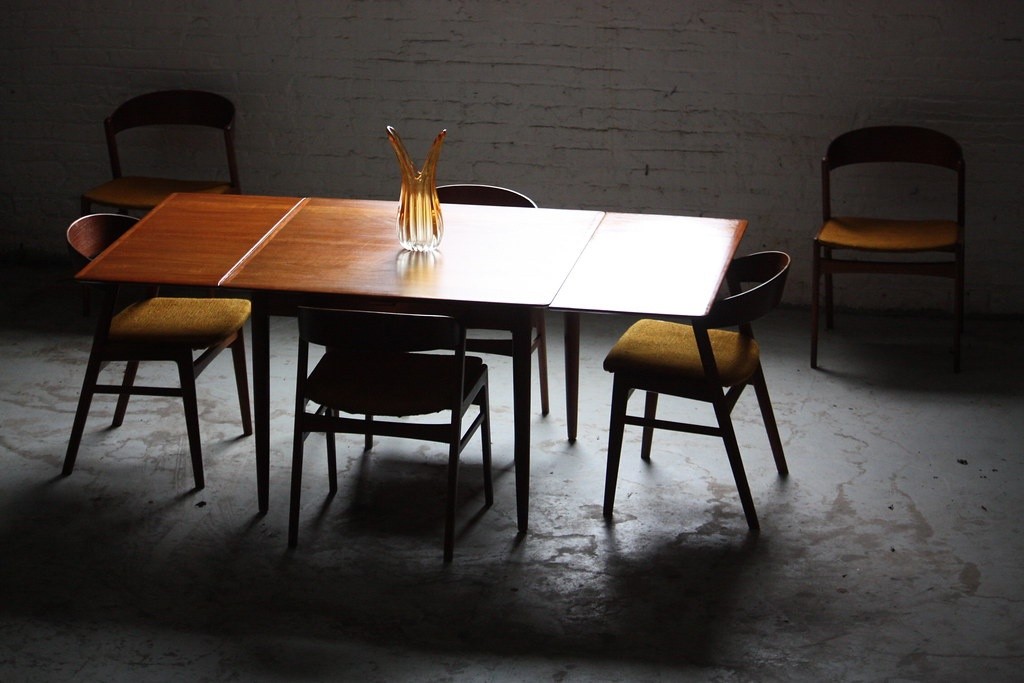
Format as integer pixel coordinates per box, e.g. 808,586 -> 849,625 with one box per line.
72,193 -> 750,533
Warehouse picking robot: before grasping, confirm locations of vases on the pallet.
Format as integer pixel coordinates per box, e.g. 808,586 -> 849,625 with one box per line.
384,125 -> 447,254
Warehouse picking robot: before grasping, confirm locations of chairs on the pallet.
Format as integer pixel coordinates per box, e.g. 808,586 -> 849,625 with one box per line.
61,213 -> 253,491
810,124 -> 967,375
286,305 -> 493,567
364,182 -> 550,451
602,250 -> 792,538
103,89 -> 258,298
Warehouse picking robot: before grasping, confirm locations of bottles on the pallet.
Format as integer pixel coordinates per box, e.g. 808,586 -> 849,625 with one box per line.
386,124 -> 448,252
